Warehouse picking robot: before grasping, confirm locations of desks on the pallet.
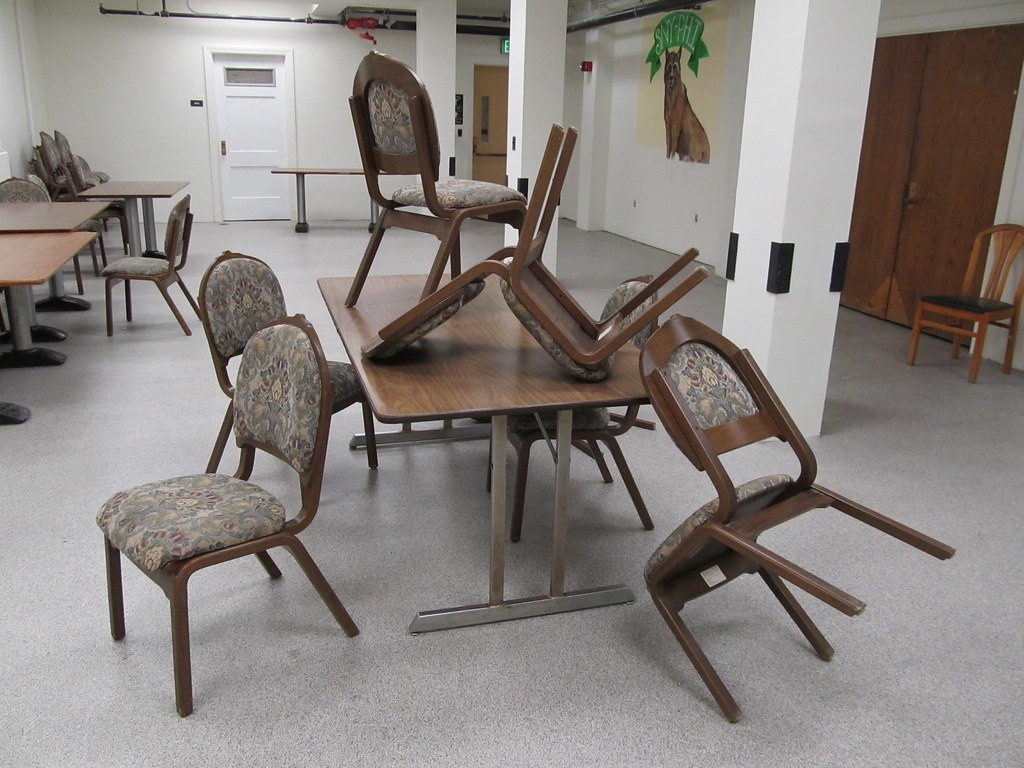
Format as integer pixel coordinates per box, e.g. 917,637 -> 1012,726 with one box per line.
316,276 -> 651,637
75,181 -> 191,259
0,201 -> 110,345
0,232 -> 99,425
270,167 -> 419,233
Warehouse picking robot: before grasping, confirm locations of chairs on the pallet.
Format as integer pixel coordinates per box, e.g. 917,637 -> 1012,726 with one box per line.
487,274 -> 659,543
97,313 -> 360,718
196,250 -> 379,474
346,49 -> 528,308
906,224 -> 1024,385
31,129 -> 130,256
362,122 -> 711,382
25,174 -> 108,295
102,194 -> 205,337
640,313 -> 957,724
0,177 -> 52,204
